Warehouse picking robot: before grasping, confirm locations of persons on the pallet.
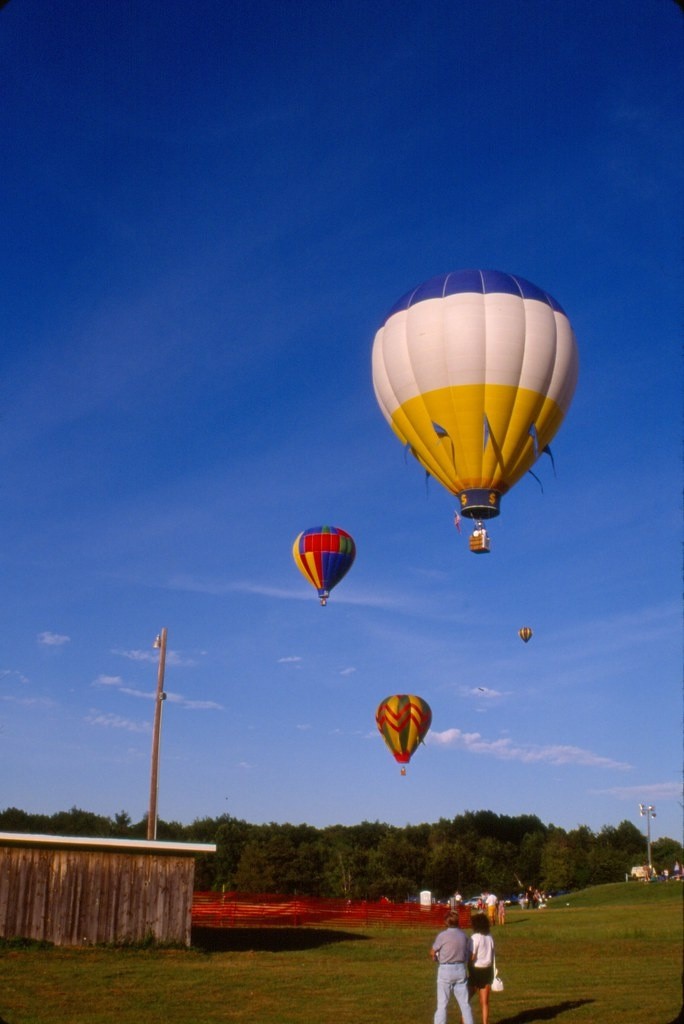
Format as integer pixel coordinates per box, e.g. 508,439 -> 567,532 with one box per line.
465,913 -> 496,1024
497,896 -> 506,925
485,890 -> 499,926
526,885 -> 541,910
643,860 -> 649,882
661,865 -> 671,882
477,890 -> 490,911
431,912 -> 474,1024
673,858 -> 680,881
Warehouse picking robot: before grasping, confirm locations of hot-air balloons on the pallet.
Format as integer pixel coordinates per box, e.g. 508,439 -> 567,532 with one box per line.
518,626 -> 534,643
375,694 -> 433,776
371,264 -> 580,555
291,525 -> 356,608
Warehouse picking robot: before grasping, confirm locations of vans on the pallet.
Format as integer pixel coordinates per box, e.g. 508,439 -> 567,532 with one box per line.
631,866 -> 656,880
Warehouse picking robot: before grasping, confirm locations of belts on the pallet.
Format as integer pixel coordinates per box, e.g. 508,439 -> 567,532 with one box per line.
440,961 -> 465,965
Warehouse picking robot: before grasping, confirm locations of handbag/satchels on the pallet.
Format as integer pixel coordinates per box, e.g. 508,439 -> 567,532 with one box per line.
491,977 -> 504,992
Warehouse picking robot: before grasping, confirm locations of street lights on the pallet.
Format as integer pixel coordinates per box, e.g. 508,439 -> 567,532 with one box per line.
639,804 -> 656,868
